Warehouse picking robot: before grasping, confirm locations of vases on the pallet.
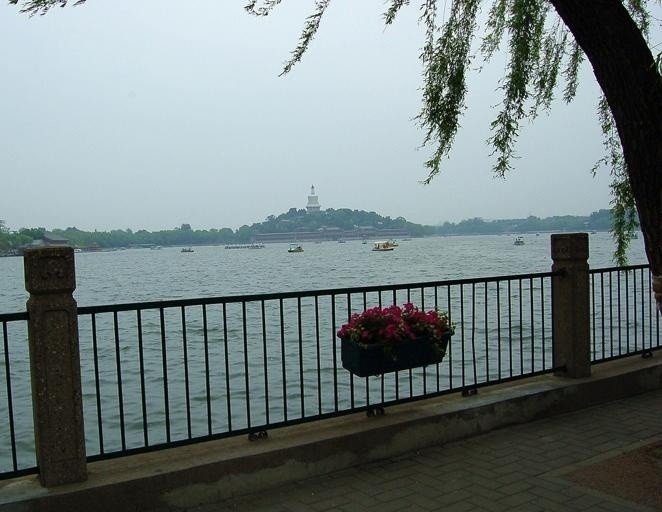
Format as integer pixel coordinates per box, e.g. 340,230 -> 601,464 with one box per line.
336,332 -> 456,378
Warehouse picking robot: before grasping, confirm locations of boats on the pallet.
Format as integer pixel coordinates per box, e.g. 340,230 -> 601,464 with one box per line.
513,235 -> 525,246
181,247 -> 194,252
624,232 -> 639,239
360,237 -> 400,252
287,242 -> 304,252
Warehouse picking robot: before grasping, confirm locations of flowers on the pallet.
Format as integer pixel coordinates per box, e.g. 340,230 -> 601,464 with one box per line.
336,301 -> 461,358
